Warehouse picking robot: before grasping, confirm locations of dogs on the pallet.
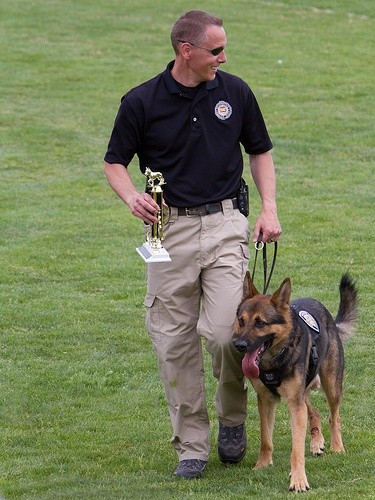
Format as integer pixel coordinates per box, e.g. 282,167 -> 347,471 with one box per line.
233,270 -> 361,493
145,167 -> 164,186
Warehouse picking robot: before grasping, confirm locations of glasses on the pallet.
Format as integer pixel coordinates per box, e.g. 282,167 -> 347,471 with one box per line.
178,40 -> 225,56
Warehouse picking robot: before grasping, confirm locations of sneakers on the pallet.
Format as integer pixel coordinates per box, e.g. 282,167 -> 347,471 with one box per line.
218,421 -> 247,464
177,459 -> 207,478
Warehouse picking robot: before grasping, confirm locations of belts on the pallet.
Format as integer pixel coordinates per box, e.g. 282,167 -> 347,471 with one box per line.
169,198 -> 238,218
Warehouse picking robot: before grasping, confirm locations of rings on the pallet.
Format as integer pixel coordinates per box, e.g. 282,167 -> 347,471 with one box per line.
270,238 -> 273,242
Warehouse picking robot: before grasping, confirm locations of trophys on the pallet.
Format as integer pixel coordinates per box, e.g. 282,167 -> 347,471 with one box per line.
134,167 -> 172,263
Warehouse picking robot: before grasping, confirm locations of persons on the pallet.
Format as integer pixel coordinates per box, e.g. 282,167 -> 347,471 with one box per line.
102,10 -> 282,478
